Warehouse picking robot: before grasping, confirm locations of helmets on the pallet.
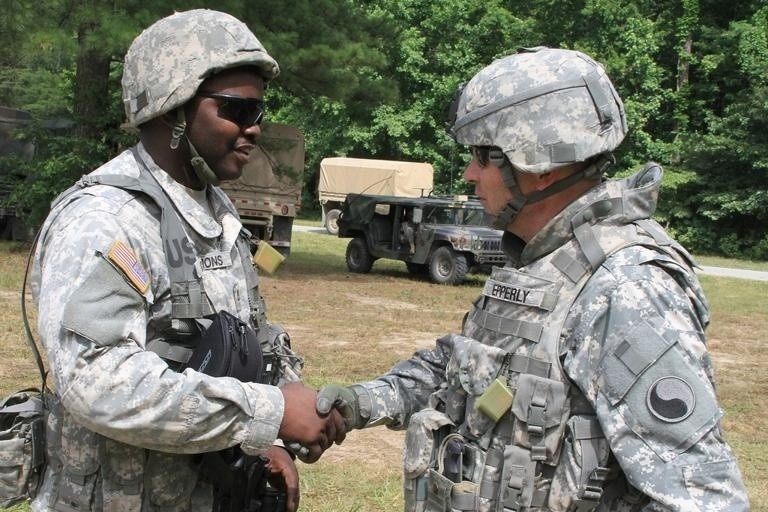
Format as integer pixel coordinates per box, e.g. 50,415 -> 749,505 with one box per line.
120,9 -> 280,132
454,46 -> 628,173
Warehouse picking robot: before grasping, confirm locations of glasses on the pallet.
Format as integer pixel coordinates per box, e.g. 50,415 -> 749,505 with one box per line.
200,90 -> 265,126
468,143 -> 493,170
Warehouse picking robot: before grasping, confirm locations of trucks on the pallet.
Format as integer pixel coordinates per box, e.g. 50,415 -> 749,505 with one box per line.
217,119 -> 306,262
316,156 -> 436,235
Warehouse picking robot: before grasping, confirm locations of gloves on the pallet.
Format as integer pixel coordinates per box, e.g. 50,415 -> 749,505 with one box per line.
282,384 -> 371,461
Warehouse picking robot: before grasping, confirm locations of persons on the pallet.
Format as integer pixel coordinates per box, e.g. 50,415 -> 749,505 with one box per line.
399,210 -> 417,254
289,45 -> 750,512
27,7 -> 347,512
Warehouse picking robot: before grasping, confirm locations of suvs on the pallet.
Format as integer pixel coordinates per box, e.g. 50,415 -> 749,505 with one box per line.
338,193 -> 510,286
